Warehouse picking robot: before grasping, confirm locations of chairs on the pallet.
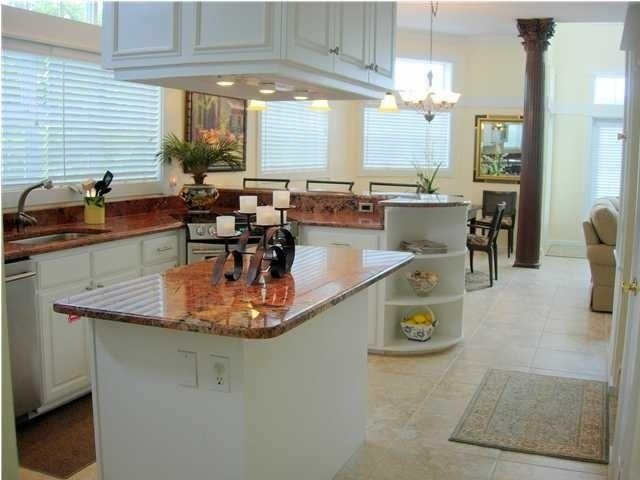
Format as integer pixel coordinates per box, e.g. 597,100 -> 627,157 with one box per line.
367,181 -> 422,198
465,202 -> 507,287
306,179 -> 353,194
242,178 -> 289,191
465,188 -> 517,259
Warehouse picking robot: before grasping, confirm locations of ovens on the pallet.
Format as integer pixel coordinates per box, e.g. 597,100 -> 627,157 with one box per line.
185,219 -> 263,264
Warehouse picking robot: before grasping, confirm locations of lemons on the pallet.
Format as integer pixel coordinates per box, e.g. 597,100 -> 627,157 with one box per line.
405,313 -> 433,326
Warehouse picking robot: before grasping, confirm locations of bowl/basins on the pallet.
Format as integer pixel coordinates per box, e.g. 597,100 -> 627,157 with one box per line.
409,278 -> 441,296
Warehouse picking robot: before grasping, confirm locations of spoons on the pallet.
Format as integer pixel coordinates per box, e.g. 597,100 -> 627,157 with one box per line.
95,181 -> 107,197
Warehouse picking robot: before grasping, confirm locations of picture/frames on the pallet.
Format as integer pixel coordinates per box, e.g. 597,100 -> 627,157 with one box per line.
472,114 -> 524,183
183,90 -> 247,175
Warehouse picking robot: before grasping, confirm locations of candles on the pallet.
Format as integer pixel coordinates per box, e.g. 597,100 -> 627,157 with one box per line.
236,195 -> 258,215
270,203 -> 289,226
252,207 -> 277,226
215,214 -> 237,238
272,187 -> 292,211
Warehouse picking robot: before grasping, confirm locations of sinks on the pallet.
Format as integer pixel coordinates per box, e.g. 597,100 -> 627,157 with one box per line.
13,232 -> 98,244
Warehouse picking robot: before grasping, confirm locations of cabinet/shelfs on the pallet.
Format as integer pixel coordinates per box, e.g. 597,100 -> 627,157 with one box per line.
11,227 -> 180,408
101,2 -> 283,68
297,223 -> 380,356
283,2 -> 395,93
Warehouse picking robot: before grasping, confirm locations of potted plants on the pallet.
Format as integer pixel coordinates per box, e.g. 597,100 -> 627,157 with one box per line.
155,130 -> 245,214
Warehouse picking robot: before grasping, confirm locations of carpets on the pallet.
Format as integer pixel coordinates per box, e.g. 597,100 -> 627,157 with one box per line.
15,392 -> 97,479
447,367 -> 610,466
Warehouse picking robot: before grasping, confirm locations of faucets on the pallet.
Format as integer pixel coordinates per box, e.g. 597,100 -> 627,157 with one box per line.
16,177 -> 53,234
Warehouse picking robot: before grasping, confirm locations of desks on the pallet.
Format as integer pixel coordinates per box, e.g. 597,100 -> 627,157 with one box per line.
52,242 -> 417,480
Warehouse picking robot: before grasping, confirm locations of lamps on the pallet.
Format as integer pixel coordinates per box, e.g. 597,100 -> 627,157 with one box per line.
378,96 -> 401,116
303,99 -> 335,114
245,98 -> 269,112
378,2 -> 462,125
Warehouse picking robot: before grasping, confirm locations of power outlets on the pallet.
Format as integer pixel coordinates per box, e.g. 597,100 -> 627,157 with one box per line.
204,351 -> 233,395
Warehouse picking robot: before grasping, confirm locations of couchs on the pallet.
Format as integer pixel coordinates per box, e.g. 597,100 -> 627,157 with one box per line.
582,196 -> 619,313
